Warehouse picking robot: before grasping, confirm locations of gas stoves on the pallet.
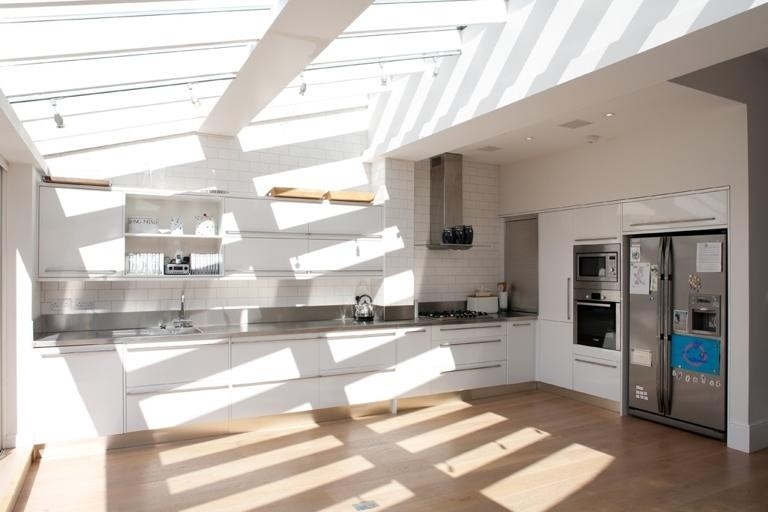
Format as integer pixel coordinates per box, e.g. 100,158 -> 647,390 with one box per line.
419,309 -> 492,323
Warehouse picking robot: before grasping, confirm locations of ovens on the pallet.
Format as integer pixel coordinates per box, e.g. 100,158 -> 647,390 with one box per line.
573,299 -> 622,351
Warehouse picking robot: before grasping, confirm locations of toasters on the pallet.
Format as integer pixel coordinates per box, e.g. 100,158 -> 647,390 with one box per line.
467,294 -> 498,315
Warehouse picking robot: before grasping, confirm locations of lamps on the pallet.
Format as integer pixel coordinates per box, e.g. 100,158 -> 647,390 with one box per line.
187,84 -> 202,108
299,72 -> 307,96
50,99 -> 64,129
433,58 -> 440,78
378,62 -> 389,85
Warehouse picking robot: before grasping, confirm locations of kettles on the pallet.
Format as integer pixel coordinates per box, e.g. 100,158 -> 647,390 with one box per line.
352,295 -> 375,321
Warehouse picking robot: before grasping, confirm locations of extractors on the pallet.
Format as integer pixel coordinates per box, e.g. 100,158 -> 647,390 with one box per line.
418,153 -> 491,250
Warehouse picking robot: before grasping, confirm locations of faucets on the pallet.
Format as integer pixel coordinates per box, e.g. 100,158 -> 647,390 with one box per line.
179,293 -> 185,320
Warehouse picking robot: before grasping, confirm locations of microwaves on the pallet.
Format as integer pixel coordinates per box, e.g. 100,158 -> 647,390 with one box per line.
572,244 -> 620,294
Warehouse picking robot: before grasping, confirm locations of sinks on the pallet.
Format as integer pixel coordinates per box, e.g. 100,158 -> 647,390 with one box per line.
169,326 -> 202,334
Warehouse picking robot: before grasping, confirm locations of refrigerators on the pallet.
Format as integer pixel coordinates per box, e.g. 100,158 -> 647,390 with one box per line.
626,235 -> 725,439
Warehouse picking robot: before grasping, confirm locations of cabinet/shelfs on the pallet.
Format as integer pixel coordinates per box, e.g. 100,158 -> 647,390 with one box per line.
400,320 -> 535,396
36,347 -> 123,437
38,185 -> 126,281
123,330 -> 398,434
222,198 -> 384,281
125,193 -> 221,280
